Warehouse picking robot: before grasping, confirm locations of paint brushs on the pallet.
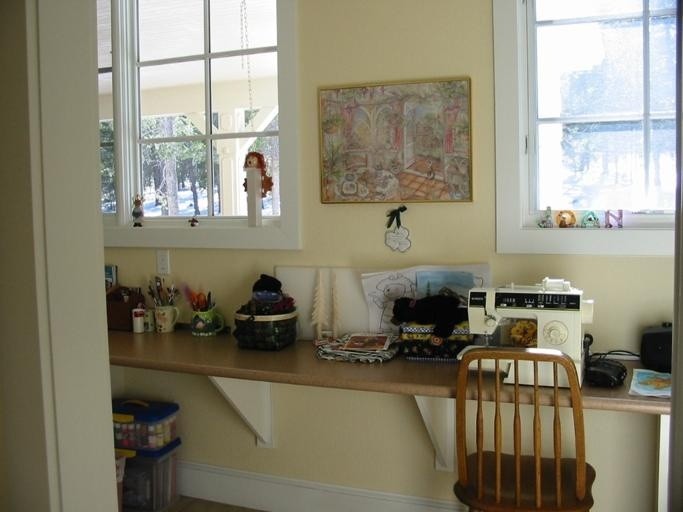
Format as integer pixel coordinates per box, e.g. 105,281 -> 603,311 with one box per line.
148,276 -> 179,306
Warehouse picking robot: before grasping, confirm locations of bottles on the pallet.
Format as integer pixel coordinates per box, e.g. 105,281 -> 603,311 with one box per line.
113,416 -> 177,450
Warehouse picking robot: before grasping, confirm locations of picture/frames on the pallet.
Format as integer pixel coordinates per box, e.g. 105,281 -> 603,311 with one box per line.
314,74 -> 475,204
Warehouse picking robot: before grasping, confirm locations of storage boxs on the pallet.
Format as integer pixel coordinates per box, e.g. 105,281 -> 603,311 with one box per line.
109,394 -> 182,510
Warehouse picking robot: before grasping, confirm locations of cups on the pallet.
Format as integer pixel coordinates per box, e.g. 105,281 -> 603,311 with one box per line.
154,306 -> 179,334
191,305 -> 224,336
131,306 -> 153,334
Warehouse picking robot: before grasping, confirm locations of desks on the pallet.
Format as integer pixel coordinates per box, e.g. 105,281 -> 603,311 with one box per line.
108,327 -> 671,511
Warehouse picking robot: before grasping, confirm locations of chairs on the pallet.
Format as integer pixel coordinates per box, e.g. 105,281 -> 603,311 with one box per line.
453,349 -> 594,511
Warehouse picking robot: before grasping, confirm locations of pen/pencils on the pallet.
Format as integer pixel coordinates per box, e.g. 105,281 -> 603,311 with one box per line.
190,290 -> 215,313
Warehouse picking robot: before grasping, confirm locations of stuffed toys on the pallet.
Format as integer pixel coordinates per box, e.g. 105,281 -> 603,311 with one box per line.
130,194 -> 146,227
389,286 -> 469,338
